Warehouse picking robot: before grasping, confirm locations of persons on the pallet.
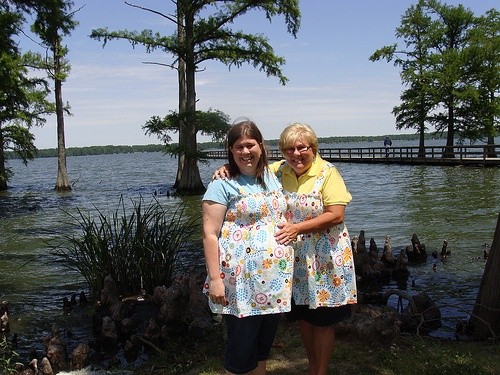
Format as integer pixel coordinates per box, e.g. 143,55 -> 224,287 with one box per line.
212,123 -> 358,375
202,117 -> 294,375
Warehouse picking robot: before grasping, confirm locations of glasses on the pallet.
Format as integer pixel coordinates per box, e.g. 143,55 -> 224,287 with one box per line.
281,145 -> 311,153
